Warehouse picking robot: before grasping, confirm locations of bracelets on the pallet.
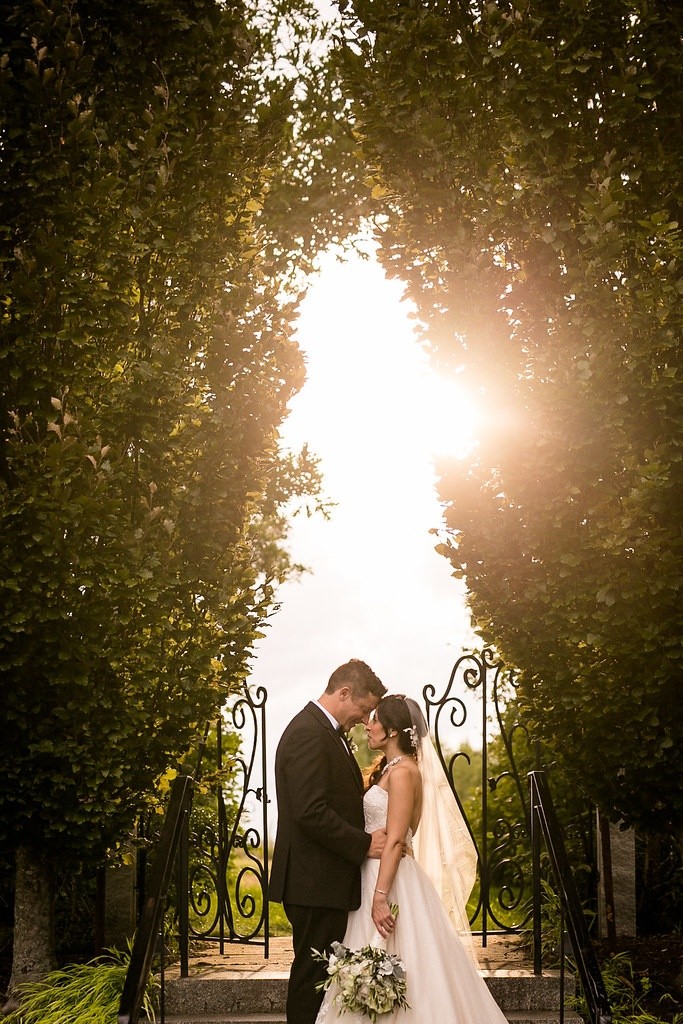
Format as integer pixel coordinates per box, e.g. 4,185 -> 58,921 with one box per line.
375,889 -> 389,896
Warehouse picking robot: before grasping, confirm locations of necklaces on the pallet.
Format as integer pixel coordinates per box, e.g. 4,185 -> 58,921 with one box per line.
380,753 -> 411,775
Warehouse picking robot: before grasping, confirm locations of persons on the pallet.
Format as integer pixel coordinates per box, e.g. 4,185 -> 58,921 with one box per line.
316,695 -> 509,1024
268,659 -> 390,1024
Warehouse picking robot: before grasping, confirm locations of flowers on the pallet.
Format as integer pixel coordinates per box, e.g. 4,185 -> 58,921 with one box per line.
310,901 -> 412,1024
346,732 -> 358,754
